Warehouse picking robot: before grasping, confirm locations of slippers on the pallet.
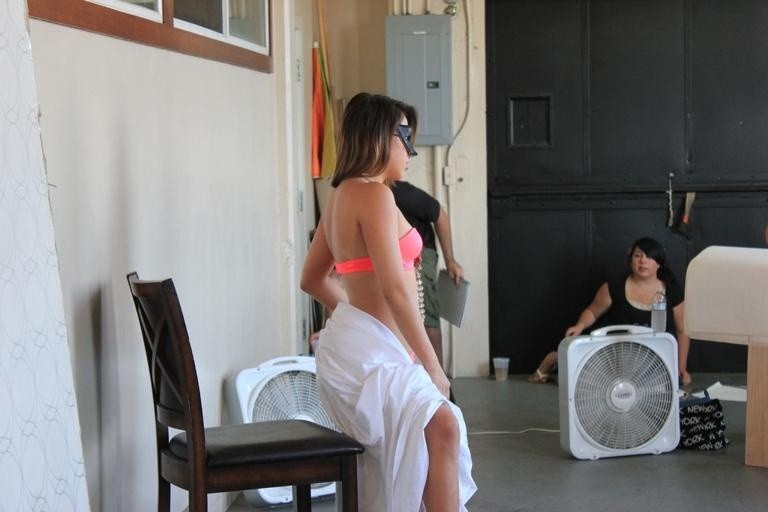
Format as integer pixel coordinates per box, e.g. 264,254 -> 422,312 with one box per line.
528,367 -> 549,384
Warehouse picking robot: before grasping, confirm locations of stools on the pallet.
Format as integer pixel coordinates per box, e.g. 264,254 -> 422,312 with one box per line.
680,244 -> 768,470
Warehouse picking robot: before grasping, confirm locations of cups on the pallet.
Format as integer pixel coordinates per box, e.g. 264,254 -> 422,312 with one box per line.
492,356 -> 511,382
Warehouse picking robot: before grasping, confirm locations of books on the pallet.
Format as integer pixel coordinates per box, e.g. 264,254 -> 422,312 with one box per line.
434,266 -> 470,327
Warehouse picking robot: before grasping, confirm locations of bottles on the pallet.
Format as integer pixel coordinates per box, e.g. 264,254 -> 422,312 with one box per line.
650,287 -> 668,334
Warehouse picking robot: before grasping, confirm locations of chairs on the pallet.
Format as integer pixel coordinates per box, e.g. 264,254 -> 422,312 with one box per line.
126,272 -> 365,512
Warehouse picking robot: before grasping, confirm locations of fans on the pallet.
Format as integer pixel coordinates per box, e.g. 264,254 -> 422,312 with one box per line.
223,354 -> 349,511
558,321 -> 681,464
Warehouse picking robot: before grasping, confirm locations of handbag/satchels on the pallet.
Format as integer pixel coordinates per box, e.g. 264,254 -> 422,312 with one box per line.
679,389 -> 731,451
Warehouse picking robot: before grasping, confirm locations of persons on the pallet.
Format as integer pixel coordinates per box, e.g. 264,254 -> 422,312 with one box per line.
525,236 -> 693,403
387,179 -> 465,405
298,90 -> 480,512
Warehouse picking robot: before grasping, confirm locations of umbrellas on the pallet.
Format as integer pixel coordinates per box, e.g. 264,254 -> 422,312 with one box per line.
306,40 -> 340,182
